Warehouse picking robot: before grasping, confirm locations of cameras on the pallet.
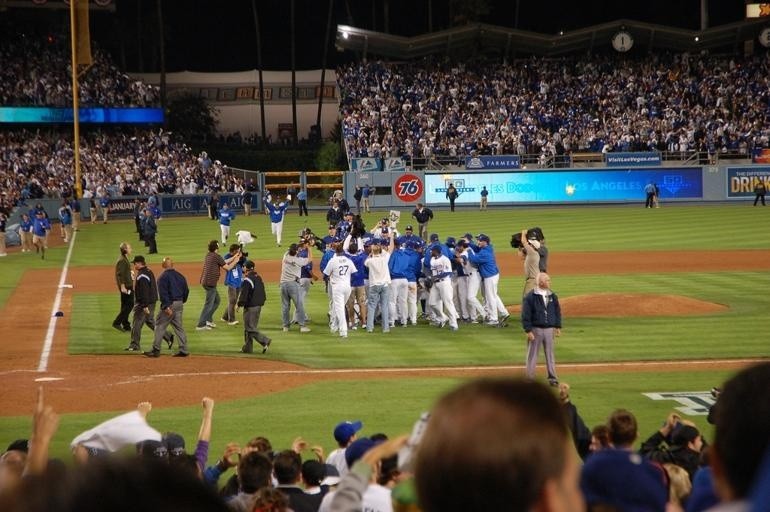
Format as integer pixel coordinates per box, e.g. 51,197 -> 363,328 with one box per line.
241,252 -> 248,257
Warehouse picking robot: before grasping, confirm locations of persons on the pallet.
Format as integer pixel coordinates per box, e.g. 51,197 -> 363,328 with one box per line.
644,180 -> 656,209
752,179 -> 767,206
649,187 -> 661,208
0,32 -> 259,260
140,257 -> 190,358
0,360 -> 770,512
123,254 -> 175,353
220,243 -> 246,325
111,243 -> 135,331
235,260 -> 272,355
195,240 -> 243,332
262,182 -> 550,341
336,46 -> 769,170
522,271 -> 563,388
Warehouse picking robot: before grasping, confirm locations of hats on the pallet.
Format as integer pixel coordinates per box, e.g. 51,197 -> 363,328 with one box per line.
667,425 -> 698,452
130,256 -> 145,263
242,260 -> 256,269
300,212 -> 490,252
344,433 -> 389,472
276,202 -> 279,205
276,196 -> 281,199
528,239 -> 540,250
334,420 -> 363,442
36,211 -> 42,215
223,203 -> 228,205
144,208 -> 152,211
136,432 -> 185,458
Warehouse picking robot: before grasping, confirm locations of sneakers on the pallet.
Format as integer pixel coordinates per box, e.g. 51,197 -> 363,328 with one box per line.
112,316 -> 312,357
550,379 -> 558,385
327,311 -> 511,338
